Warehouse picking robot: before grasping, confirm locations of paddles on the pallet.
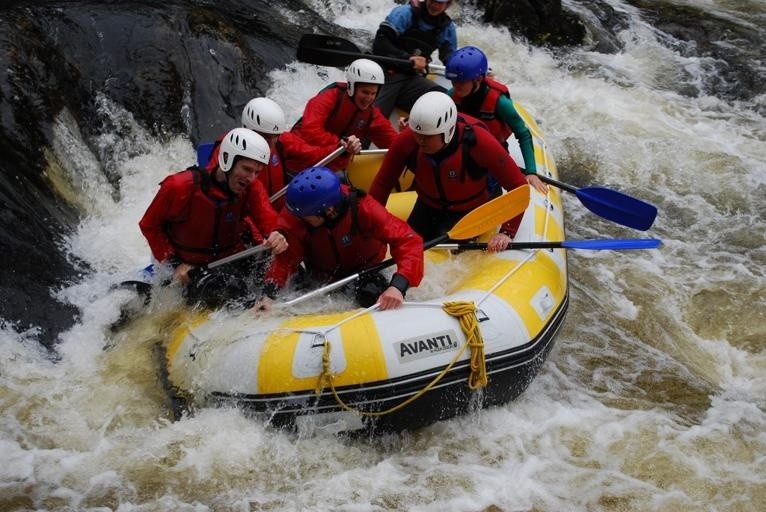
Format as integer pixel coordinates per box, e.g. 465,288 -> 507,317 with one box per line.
296,183 -> 530,306
519,167 -> 657,230
297,34 -> 444,70
102,246 -> 264,328
444,239 -> 657,249
197,143 -> 386,170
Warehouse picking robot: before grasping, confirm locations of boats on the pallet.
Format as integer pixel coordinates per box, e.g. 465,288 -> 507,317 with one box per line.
152,70 -> 570,447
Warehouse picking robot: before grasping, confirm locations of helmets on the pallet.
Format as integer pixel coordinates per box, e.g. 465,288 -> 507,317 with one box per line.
347,59 -> 385,96
409,46 -> 488,144
286,167 -> 341,216
218,97 -> 284,172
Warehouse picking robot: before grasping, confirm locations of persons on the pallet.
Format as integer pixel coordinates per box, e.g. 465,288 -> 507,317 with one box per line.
399,45 -> 549,196
136,127 -> 288,308
207,57 -> 399,211
363,1 -> 457,150
254,166 -> 423,309
369,91 -> 530,252
